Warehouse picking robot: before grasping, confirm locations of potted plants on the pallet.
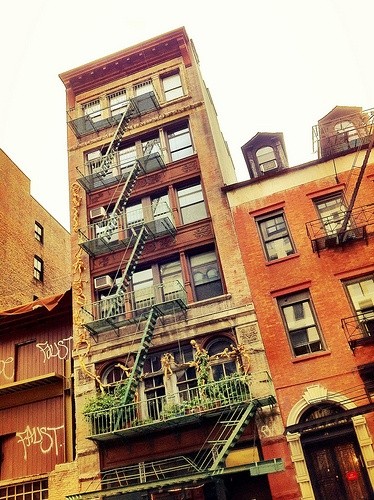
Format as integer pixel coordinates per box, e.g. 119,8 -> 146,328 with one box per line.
122,396 -> 221,429
